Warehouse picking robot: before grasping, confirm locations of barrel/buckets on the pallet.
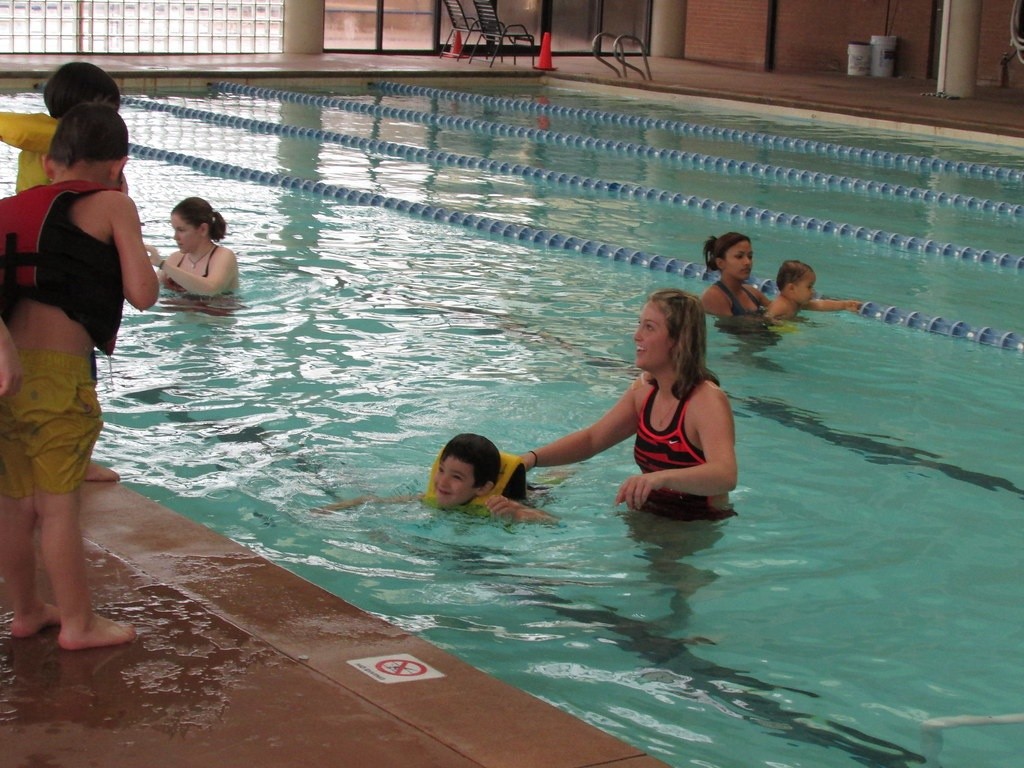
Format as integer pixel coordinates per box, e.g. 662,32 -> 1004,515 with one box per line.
847,42 -> 873,75
870,36 -> 896,76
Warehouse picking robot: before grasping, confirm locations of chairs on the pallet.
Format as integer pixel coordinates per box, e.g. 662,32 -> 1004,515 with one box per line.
439,0 -> 504,63
467,0 -> 535,69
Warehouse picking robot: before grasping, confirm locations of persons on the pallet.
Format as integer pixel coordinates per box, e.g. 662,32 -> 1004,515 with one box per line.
0,61 -> 160,651
697,232 -> 771,315
765,260 -> 861,318
519,288 -> 738,508
145,196 -> 239,296
434,433 -> 510,515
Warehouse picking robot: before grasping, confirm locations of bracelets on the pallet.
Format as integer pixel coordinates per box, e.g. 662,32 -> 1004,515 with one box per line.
159,260 -> 164,269
530,451 -> 538,467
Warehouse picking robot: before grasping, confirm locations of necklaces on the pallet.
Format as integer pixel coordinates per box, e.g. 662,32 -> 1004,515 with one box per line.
188,246 -> 212,268
